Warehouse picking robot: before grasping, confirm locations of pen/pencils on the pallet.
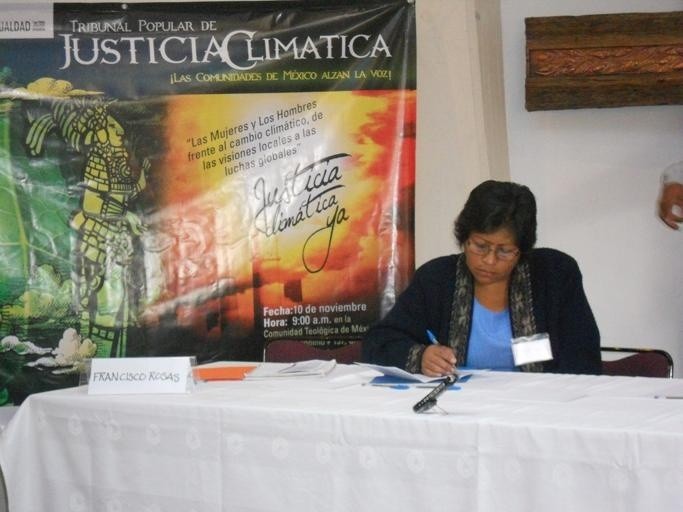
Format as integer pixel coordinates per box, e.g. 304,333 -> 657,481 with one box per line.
426,329 -> 457,369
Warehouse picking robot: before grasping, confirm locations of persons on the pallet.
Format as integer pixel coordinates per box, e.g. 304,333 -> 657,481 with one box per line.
364,180 -> 605,379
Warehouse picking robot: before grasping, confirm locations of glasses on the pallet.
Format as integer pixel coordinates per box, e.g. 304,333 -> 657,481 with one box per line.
466,236 -> 521,262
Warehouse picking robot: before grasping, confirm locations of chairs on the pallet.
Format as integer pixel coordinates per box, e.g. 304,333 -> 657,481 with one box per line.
594,345 -> 673,377
261,337 -> 363,364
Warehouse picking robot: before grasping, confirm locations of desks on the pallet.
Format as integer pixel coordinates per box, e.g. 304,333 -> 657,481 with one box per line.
0,361 -> 682,512
